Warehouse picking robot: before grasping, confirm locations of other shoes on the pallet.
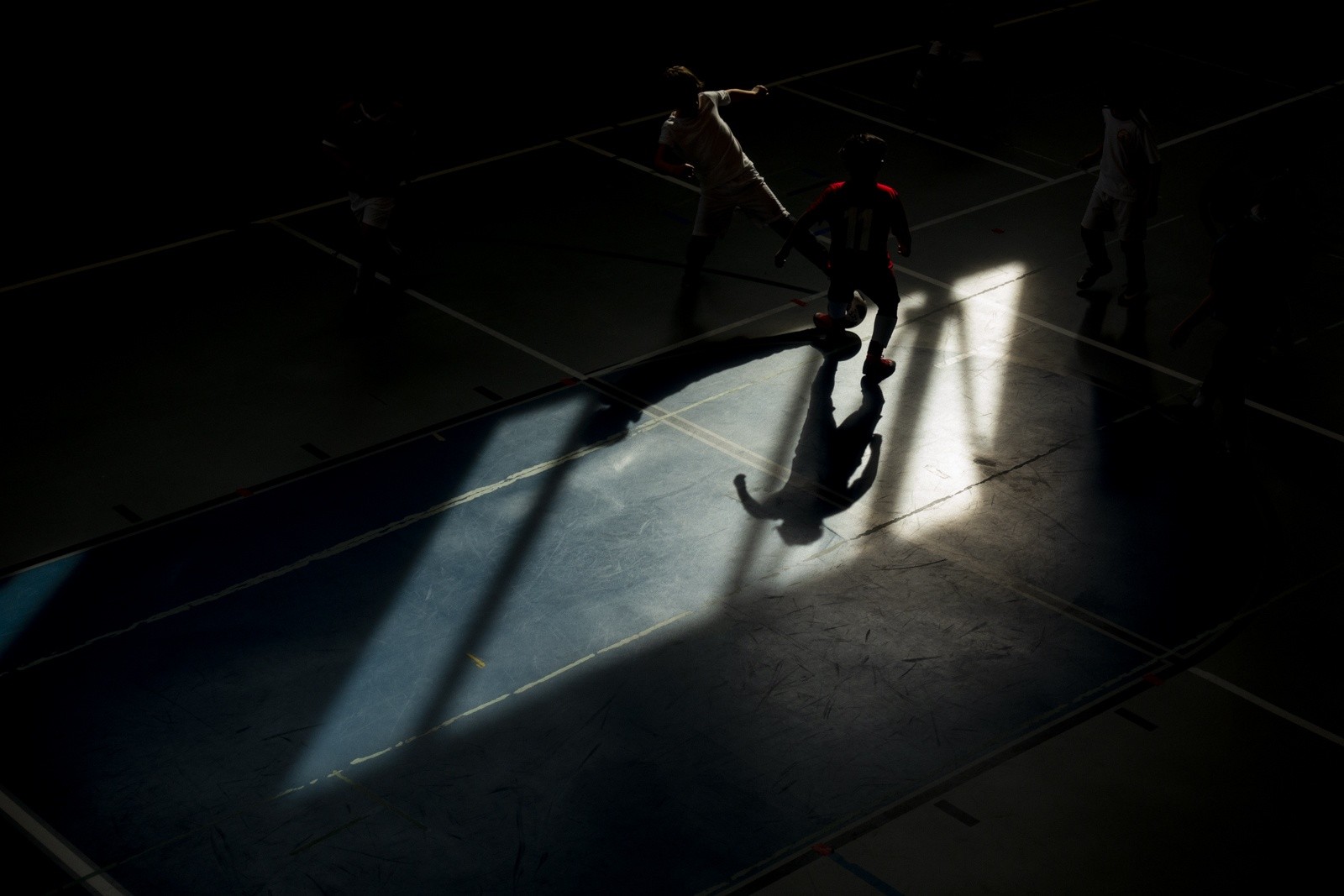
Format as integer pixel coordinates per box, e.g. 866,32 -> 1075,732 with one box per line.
1122,287 -> 1141,299
863,357 -> 896,375
813,313 -> 845,338
1076,260 -> 1112,288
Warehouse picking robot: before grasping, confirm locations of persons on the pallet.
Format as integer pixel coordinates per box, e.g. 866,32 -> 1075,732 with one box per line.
654,65 -> 830,281
774,133 -> 911,374
1169,180 -> 1277,453
323,70 -> 421,320
1076,78 -> 1160,296
